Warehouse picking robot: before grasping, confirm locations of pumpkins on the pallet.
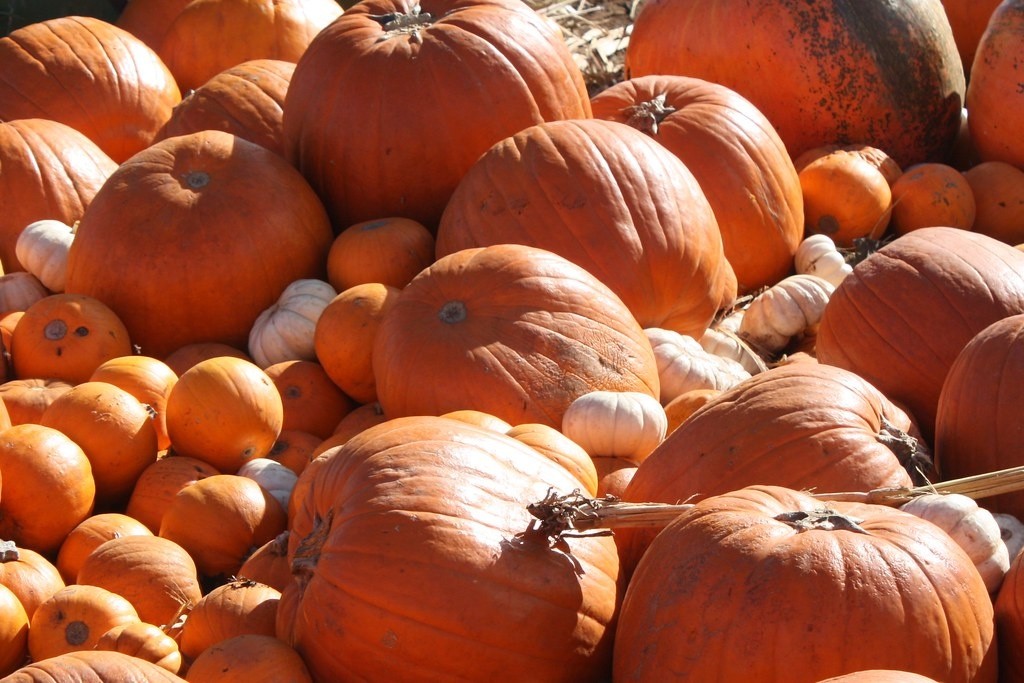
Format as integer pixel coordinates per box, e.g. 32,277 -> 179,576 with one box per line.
0,0 -> 1024,683
